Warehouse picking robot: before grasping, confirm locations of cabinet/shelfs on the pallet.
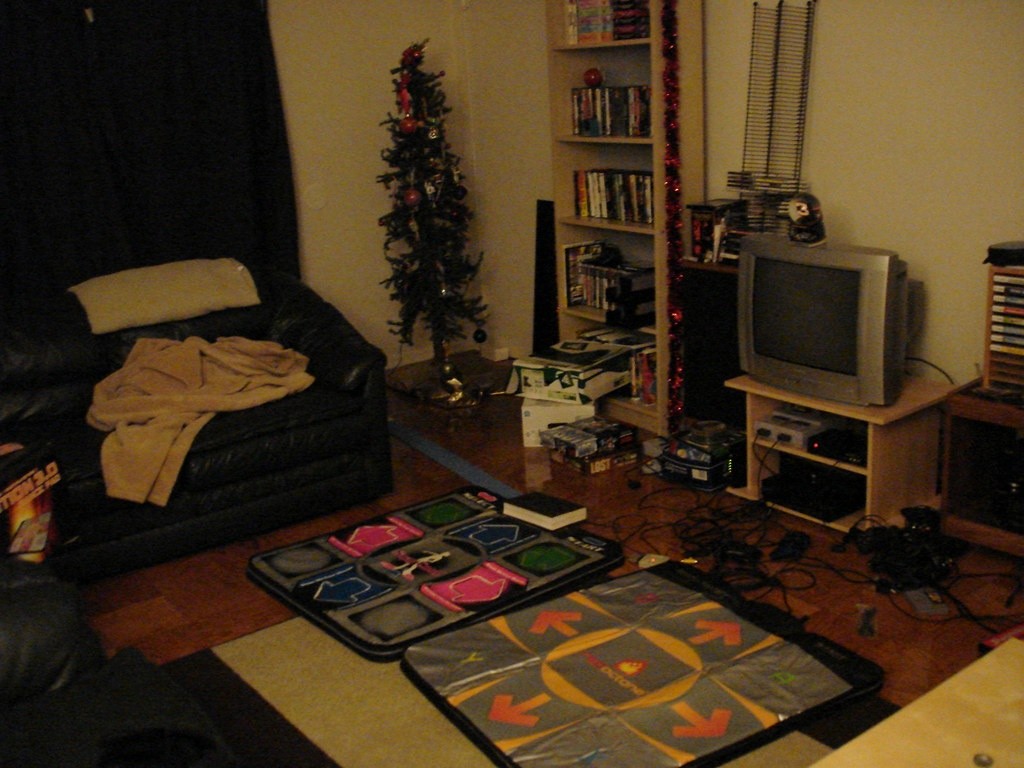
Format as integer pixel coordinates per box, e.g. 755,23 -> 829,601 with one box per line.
723,373 -> 959,535
936,267 -> 1024,557
547,0 -> 707,439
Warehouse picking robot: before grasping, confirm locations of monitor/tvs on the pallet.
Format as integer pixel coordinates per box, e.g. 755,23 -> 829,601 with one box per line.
736,232 -> 926,408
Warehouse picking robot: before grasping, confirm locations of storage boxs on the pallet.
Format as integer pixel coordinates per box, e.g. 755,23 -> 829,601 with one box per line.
503,339 -> 641,472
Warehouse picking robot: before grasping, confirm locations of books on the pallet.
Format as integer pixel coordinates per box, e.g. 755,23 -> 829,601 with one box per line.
569,86 -> 650,137
562,238 -> 655,317
574,169 -> 653,224
989,275 -> 1024,355
575,329 -> 657,409
500,490 -> 587,533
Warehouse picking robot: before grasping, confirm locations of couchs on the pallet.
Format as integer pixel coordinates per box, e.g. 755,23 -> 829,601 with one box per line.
0,258 -> 393,581
